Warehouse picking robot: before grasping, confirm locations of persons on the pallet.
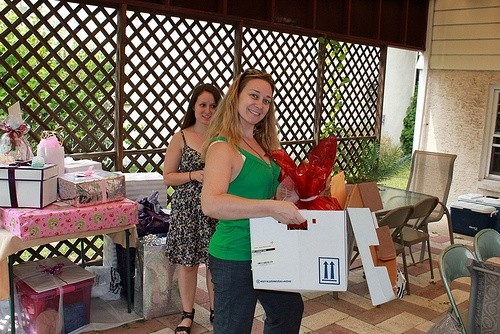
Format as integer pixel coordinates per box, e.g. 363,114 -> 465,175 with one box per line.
162,83 -> 222,334
198,68 -> 308,334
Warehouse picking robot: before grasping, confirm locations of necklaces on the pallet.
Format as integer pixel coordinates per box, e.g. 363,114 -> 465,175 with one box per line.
239,135 -> 269,163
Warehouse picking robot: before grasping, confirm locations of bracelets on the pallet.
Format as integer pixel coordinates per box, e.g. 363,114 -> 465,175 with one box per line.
189,171 -> 192,180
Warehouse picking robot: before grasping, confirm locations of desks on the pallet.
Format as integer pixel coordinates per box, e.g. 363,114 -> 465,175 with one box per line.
0,224 -> 135,334
376,184 -> 434,264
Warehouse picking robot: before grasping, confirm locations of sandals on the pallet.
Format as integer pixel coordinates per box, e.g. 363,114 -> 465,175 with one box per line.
209,307 -> 215,325
174,308 -> 196,334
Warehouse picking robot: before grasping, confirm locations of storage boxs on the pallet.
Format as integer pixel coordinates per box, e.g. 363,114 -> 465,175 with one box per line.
249,181 -> 398,305
449,201 -> 498,237
456,193 -> 500,233
12,257 -> 95,334
0,160 -> 138,240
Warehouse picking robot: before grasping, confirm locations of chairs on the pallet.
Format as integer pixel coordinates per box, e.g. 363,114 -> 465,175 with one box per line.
332,151 -> 500,334
